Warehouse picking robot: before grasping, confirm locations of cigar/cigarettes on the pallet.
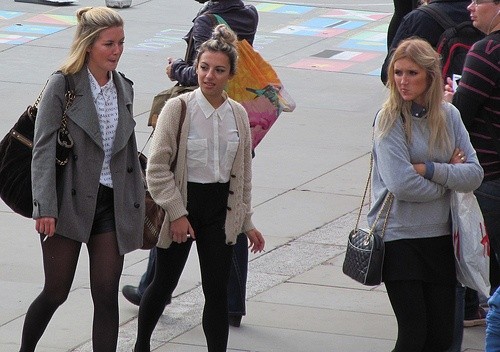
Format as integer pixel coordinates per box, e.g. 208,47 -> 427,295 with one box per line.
43,234 -> 49,242
262,250 -> 266,252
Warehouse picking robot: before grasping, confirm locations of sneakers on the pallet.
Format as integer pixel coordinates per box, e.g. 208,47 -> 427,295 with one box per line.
462,308 -> 491,327
122,285 -> 144,306
228,315 -> 242,326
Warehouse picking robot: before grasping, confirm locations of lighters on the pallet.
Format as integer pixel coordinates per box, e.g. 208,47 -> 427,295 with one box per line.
187,234 -> 190,238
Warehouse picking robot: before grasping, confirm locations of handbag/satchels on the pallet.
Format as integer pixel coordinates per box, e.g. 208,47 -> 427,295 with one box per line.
207,13 -> 296,152
342,228 -> 385,286
147,10 -> 217,126
0,70 -> 76,218
137,98 -> 187,250
449,190 -> 491,299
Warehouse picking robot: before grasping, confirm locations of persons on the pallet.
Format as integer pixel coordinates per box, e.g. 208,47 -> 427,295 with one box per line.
381,0 -> 487,101
132,24 -> 265,352
367,36 -> 484,352
444,0 -> 500,327
122,0 -> 259,327
20,7 -> 146,352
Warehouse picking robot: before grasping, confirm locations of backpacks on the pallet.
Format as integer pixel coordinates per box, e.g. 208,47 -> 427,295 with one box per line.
415,4 -> 487,86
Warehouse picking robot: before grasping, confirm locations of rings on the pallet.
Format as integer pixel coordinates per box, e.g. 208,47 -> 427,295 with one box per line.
458,153 -> 462,157
461,157 -> 465,161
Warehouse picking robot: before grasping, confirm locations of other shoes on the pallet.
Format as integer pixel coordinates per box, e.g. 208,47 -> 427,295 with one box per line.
251,149 -> 255,158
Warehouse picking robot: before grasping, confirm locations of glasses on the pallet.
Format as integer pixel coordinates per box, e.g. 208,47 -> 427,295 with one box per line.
472,0 -> 500,7
